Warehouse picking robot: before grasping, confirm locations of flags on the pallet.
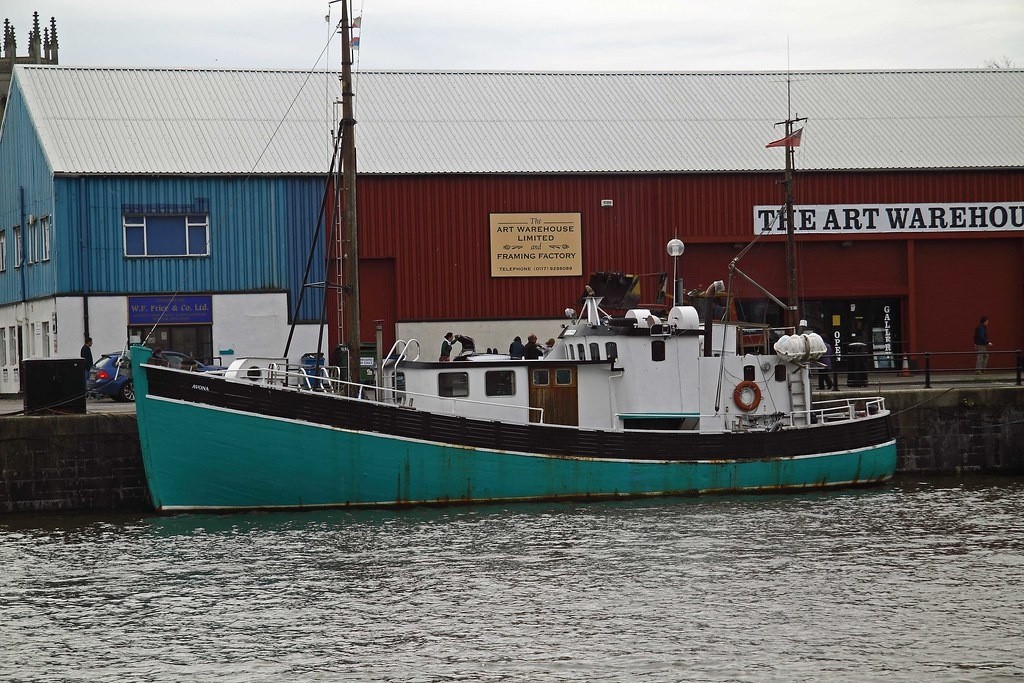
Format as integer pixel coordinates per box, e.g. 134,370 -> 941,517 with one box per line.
766,127 -> 804,148
350,16 -> 361,29
348,37 -> 359,49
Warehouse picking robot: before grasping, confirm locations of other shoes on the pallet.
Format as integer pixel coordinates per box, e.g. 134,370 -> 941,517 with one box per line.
816,388 -> 825,390
87,393 -> 96,398
827,384 -> 833,390
975,371 -> 989,375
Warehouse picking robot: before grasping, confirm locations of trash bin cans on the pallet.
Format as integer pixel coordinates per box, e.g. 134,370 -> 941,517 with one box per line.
301,352 -> 325,385
335,343 -> 379,389
846,341 -> 870,386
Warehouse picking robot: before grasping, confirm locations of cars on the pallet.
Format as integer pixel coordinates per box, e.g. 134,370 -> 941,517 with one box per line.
89,351 -> 229,402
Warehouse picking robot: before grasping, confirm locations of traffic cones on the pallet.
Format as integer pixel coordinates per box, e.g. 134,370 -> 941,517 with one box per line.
897,351 -> 913,377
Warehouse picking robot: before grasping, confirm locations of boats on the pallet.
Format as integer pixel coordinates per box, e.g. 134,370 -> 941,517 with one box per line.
129,0 -> 897,515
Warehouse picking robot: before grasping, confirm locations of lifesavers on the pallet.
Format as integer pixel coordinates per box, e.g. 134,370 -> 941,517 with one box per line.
733,381 -> 761,412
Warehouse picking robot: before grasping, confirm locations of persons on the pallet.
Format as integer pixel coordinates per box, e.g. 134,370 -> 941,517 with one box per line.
538,338 -> 555,355
973,316 -> 993,375
509,336 -> 525,360
80,337 -> 93,382
439,332 -> 459,361
524,334 -> 543,359
815,334 -> 834,390
